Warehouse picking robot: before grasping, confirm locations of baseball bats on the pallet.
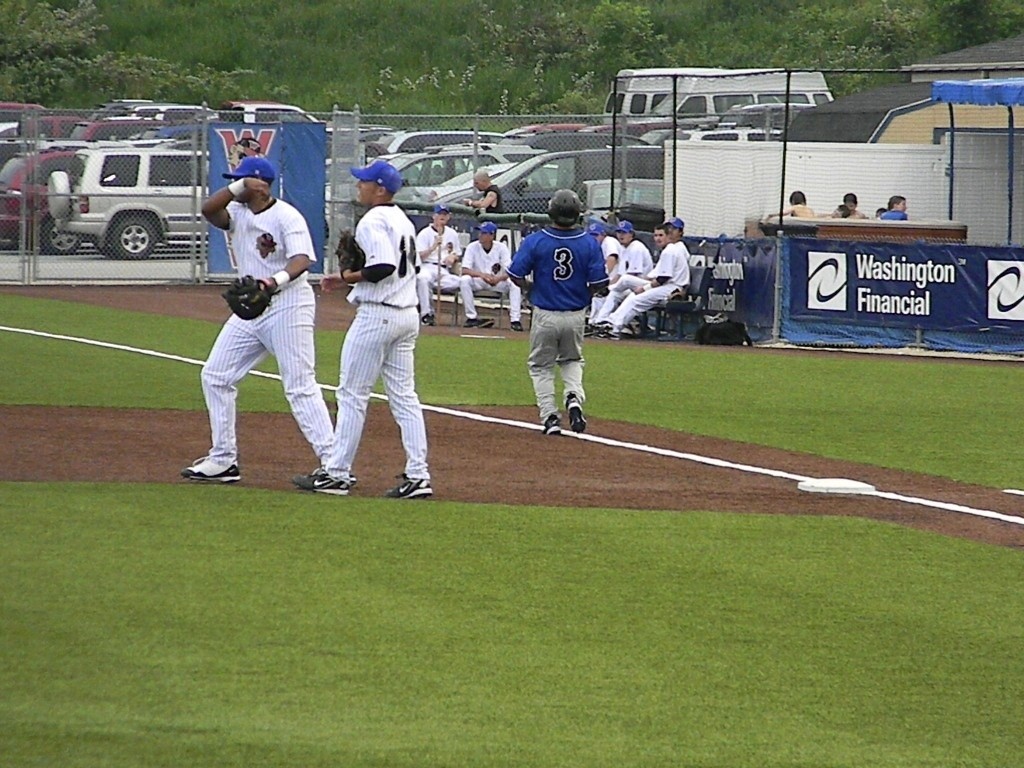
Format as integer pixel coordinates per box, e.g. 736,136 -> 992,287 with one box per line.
452,263 -> 462,326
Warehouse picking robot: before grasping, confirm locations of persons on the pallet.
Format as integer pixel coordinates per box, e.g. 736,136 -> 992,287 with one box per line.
454,171 -> 504,213
761,192 -> 907,228
290,161 -> 433,498
583,217 -> 690,340
416,205 -> 524,331
505,188 -> 608,434
181,156 -> 357,486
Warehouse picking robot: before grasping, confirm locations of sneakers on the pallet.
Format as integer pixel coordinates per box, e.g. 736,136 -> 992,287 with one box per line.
565,392 -> 587,433
511,321 -> 523,331
386,478 -> 434,499
543,415 -> 562,435
293,473 -> 350,495
422,314 -> 434,325
182,457 -> 241,482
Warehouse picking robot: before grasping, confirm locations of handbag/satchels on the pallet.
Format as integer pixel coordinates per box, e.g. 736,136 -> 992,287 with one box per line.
696,319 -> 754,346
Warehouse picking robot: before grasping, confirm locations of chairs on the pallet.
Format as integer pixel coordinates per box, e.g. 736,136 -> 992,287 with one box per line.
448,247 -> 715,343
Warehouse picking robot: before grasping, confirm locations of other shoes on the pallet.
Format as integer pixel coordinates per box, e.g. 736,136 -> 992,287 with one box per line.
463,318 -> 480,327
584,325 -> 596,336
592,329 -> 621,341
590,318 -> 614,331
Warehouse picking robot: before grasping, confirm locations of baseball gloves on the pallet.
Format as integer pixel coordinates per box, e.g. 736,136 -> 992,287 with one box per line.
333,232 -> 366,279
225,275 -> 274,320
478,319 -> 495,328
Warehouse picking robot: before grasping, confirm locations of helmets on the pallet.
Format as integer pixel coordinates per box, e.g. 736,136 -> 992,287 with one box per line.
547,189 -> 580,227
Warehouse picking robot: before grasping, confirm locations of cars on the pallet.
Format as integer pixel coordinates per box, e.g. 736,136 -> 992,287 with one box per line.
0,65 -> 837,262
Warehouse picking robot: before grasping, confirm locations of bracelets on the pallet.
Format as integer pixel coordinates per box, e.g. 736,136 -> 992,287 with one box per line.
271,270 -> 289,292
227,178 -> 246,197
469,201 -> 473,205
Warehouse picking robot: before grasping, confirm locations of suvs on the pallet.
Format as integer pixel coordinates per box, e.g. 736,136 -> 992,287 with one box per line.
45,144 -> 237,262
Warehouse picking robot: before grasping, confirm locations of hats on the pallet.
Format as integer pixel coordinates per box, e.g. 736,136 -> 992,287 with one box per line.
474,221 -> 496,232
349,160 -> 401,193
587,223 -> 605,234
434,204 -> 450,213
614,220 -> 633,232
663,217 -> 684,227
222,156 -> 275,179
